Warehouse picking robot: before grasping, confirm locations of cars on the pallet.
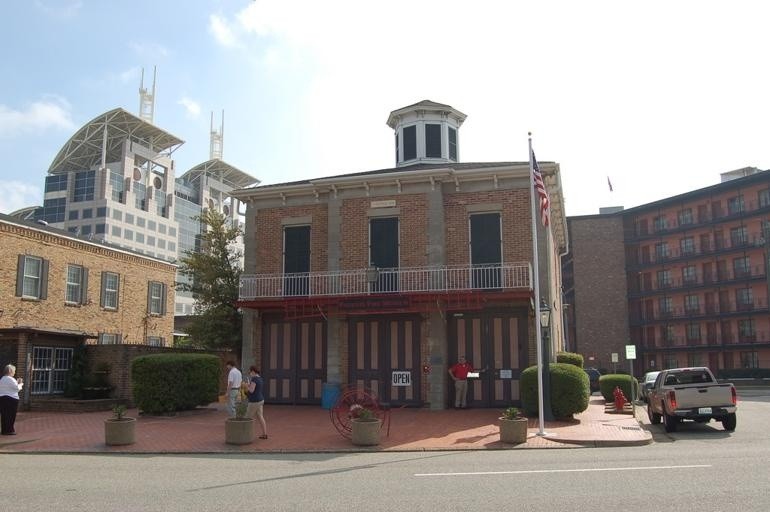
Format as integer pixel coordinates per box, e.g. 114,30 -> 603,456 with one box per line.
638,371 -> 661,404
582,368 -> 602,395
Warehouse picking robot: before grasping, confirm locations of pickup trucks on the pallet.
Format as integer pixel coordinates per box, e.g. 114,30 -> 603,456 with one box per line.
648,367 -> 737,434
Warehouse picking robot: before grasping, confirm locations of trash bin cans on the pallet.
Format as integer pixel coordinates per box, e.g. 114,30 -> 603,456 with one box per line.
322,383 -> 341,409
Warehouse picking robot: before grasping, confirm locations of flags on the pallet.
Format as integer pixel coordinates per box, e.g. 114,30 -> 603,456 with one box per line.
531,150 -> 552,231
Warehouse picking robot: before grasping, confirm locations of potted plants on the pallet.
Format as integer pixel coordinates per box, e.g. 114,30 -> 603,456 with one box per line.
104,403 -> 136,445
351,410 -> 382,445
498,407 -> 528,443
225,401 -> 256,444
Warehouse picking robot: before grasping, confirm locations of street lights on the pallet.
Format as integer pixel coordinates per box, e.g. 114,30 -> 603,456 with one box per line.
539,295 -> 555,423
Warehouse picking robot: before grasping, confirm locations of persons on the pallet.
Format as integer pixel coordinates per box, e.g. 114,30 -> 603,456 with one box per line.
242,364 -> 268,439
448,355 -> 475,410
224,360 -> 242,420
0,363 -> 24,435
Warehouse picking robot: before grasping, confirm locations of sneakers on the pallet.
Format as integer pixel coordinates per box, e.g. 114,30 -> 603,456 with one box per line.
0,431 -> 16,435
259,434 -> 267,439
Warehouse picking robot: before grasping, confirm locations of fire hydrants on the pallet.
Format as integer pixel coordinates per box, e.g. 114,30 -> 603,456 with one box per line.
614,386 -> 627,414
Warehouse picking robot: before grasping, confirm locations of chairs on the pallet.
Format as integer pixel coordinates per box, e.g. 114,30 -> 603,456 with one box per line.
666,376 -> 678,384
691,375 -> 704,382
